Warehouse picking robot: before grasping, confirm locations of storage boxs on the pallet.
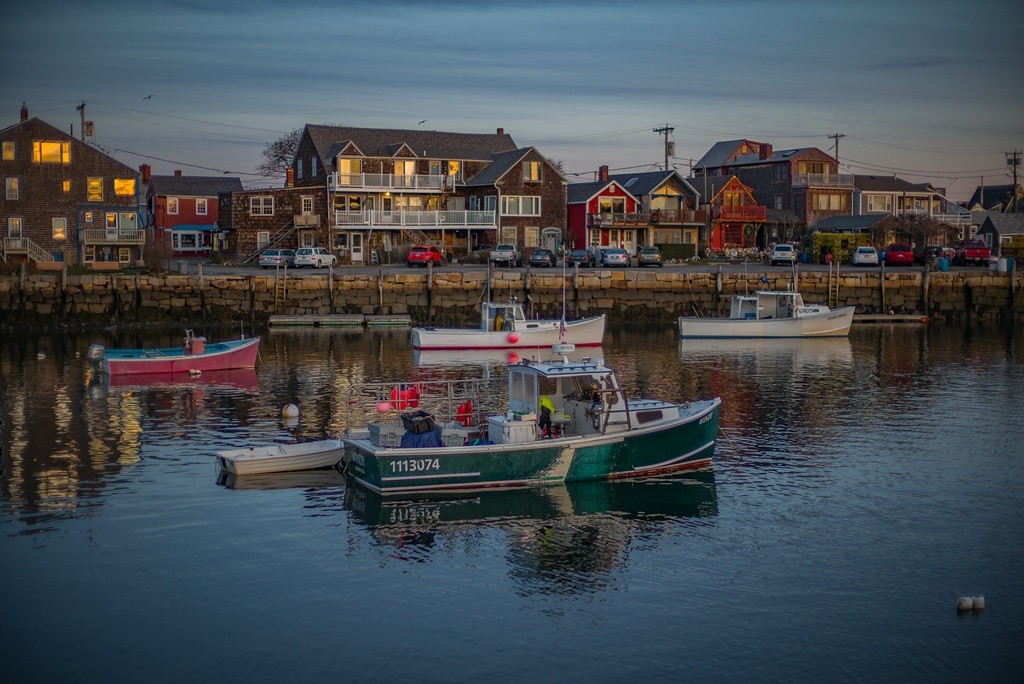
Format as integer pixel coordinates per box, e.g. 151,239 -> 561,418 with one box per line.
487,415 -> 536,444
401,410 -> 436,434
441,429 -> 467,447
368,423 -> 405,447
514,414 -> 537,421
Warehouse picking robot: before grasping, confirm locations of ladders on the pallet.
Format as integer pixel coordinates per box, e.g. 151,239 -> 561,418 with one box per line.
275,263 -> 288,316
828,260 -> 839,308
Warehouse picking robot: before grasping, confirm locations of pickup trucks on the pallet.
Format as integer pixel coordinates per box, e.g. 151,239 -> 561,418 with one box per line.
952,240 -> 991,267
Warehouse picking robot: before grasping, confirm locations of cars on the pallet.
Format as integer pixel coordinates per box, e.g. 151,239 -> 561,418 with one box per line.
638,246 -> 664,267
603,248 -> 632,268
850,247 -> 878,266
406,246 -> 444,267
918,244 -> 943,265
568,249 -> 596,268
885,243 -> 913,266
528,248 -> 557,268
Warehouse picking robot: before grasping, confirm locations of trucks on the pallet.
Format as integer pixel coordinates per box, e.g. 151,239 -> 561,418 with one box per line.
771,244 -> 795,266
491,243 -> 522,268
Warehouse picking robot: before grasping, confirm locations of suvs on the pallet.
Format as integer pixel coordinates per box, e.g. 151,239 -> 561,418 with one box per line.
293,247 -> 338,268
259,248 -> 296,268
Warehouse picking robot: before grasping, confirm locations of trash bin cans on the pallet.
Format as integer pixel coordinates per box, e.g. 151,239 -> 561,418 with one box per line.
601,249 -> 608,262
989,257 -> 1015,272
938,259 -> 947,272
448,252 -> 452,262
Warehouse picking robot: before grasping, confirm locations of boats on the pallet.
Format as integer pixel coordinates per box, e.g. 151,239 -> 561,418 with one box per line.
342,250 -> 722,497
98,368 -> 262,398
409,256 -> 606,351
678,290 -> 856,338
88,321 -> 260,376
216,439 -> 344,475
216,467 -> 346,492
680,339 -> 851,364
411,345 -> 605,371
348,465 -> 717,528
